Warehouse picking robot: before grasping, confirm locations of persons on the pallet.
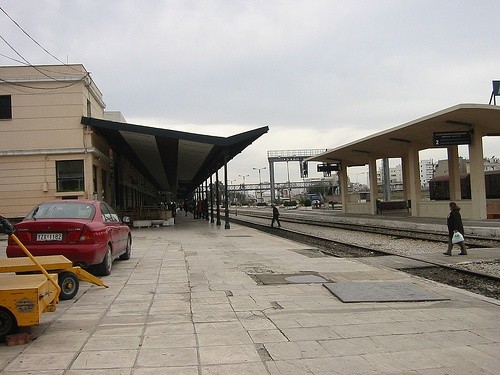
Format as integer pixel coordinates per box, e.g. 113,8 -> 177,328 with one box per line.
443,202 -> 467,255
168,199 -> 208,219
270,204 -> 281,227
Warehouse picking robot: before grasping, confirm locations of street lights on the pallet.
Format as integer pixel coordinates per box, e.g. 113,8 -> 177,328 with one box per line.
239,174 -> 250,184
227,179 -> 236,185
252,167 -> 267,204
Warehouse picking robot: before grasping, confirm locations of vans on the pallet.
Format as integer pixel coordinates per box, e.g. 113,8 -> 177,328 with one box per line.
280,197 -> 291,204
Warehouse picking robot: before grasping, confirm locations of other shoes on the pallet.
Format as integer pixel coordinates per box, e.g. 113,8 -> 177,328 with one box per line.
443,251 -> 451,255
458,251 -> 467,255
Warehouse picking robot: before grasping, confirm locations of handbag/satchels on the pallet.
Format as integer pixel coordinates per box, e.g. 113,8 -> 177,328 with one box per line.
451,231 -> 465,245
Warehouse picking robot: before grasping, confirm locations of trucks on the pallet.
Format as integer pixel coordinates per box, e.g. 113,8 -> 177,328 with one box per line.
303,193 -> 322,205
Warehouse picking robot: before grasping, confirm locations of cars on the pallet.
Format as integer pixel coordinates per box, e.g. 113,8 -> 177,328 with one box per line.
6,198 -> 132,277
295,199 -> 301,204
